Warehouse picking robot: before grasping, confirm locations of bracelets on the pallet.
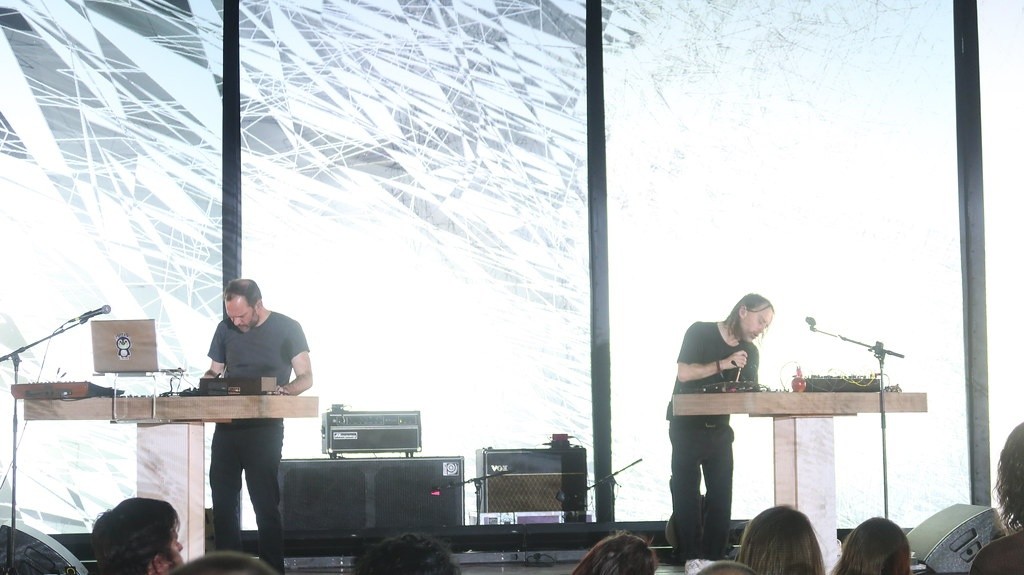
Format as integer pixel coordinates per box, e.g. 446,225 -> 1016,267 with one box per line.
716,359 -> 722,374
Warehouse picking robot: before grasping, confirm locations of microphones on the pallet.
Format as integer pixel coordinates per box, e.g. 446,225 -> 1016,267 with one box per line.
75,304 -> 111,319
734,368 -> 742,382
557,490 -> 571,501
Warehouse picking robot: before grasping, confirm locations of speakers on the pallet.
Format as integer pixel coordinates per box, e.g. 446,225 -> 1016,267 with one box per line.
906,502 -> 1006,573
0,519 -> 88,575
277,455 -> 465,531
476,449 -> 587,526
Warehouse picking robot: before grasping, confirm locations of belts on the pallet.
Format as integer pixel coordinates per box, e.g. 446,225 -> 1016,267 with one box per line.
672,419 -> 729,430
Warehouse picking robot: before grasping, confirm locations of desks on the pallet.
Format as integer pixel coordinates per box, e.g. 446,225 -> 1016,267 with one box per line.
22,395 -> 319,575
672,392 -> 927,575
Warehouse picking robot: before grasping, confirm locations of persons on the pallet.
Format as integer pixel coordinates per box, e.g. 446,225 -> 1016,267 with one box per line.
571,533 -> 659,575
202,279 -> 312,573
665,294 -> 774,561
90,498 -> 185,575
827,517 -> 912,575
170,550 -> 281,575
969,422 -> 1024,575
733,506 -> 826,575
353,532 -> 462,575
695,561 -> 758,575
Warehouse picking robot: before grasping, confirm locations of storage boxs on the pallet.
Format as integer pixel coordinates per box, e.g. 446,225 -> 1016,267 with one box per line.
277,457 -> 468,533
474,446 -> 589,528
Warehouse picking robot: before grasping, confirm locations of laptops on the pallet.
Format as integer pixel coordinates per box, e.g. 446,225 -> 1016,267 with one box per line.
91,318 -> 185,373
